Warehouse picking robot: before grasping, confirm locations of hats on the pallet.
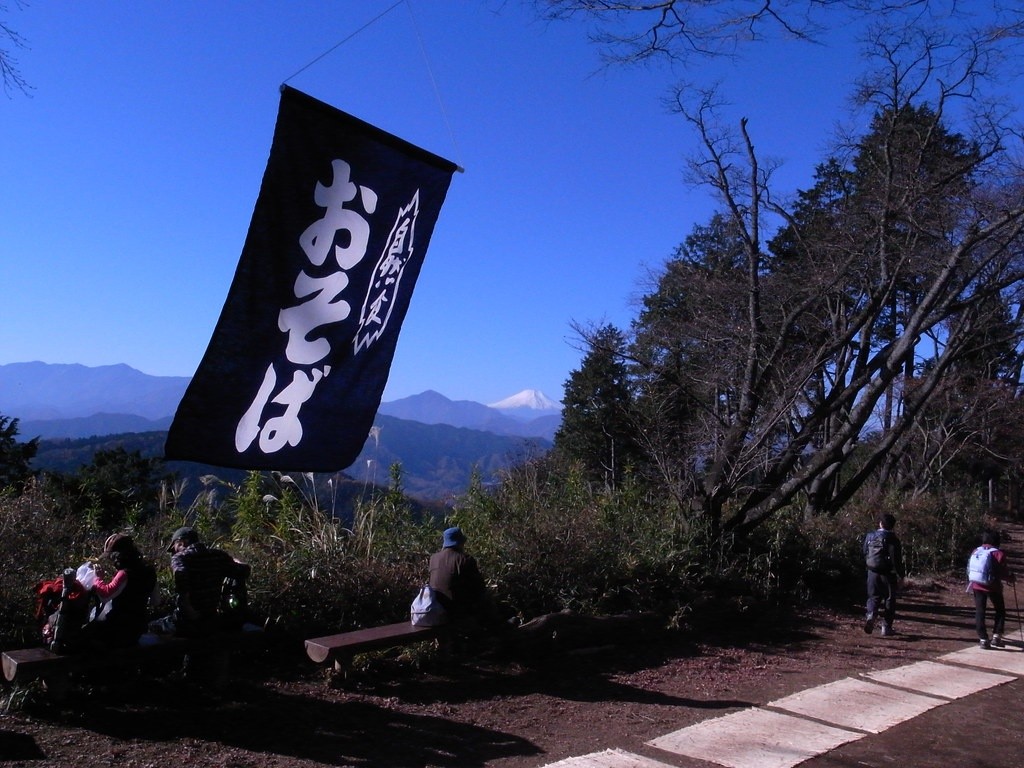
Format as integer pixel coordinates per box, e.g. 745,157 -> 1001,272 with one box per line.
98,532 -> 134,560
166,526 -> 199,552
442,527 -> 467,548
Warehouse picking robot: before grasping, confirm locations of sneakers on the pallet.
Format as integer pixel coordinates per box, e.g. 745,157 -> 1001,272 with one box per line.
882,626 -> 895,635
980,638 -> 991,648
864,614 -> 875,634
991,634 -> 1005,647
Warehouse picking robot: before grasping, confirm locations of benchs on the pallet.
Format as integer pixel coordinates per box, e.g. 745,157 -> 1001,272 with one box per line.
305,621 -> 452,692
1,623 -> 265,709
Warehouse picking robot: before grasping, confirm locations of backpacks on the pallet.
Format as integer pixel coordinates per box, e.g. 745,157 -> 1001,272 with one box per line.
865,532 -> 894,573
32,561 -> 107,650
967,546 -> 998,585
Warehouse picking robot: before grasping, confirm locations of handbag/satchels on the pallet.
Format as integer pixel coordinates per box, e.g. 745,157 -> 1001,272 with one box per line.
410,583 -> 460,627
216,574 -> 249,615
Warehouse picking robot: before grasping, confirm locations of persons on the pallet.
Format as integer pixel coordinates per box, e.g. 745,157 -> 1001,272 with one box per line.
863,513 -> 906,636
428,529 -> 487,626
48,532 -> 160,683
966,529 -> 1015,649
148,527 -> 251,637
